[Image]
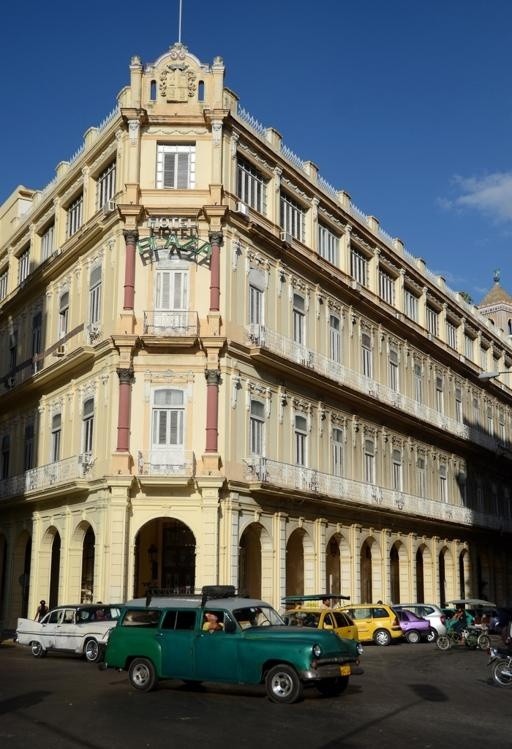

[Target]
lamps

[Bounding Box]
[147,544,159,561]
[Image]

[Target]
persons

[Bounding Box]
[321,598,331,609]
[35,600,50,623]
[93,601,109,622]
[375,601,386,616]
[452,606,468,639]
[202,611,224,632]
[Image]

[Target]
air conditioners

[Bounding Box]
[4,324,99,391]
[102,200,115,214]
[52,248,61,258]
[279,232,293,245]
[78,452,92,465]
[352,282,362,294]
[235,203,250,217]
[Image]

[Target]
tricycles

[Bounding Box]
[436,626,491,651]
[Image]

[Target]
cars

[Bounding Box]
[389,603,447,643]
[15,603,124,664]
[437,606,511,636]
[281,607,359,643]
[103,585,365,705]
[335,604,402,647]
[385,609,432,644]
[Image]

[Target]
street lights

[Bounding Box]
[475,370,512,380]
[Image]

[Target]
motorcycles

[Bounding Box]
[485,645,512,688]
